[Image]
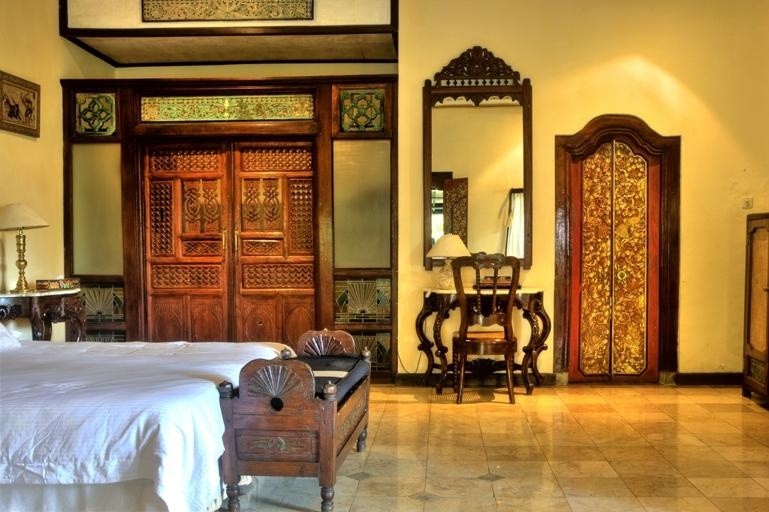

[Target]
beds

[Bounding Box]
[0,325,296,512]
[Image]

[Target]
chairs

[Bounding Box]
[450,252,520,406]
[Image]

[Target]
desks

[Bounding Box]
[416,288,551,395]
[0,289,86,343]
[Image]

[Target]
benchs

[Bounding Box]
[219,329,371,512]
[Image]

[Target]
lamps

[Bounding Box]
[425,233,472,289]
[0,205,49,293]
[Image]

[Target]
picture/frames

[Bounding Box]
[0,68,41,140]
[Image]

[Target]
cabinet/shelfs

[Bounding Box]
[554,115,682,388]
[741,212,768,410]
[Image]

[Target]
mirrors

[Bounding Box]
[422,46,533,272]
